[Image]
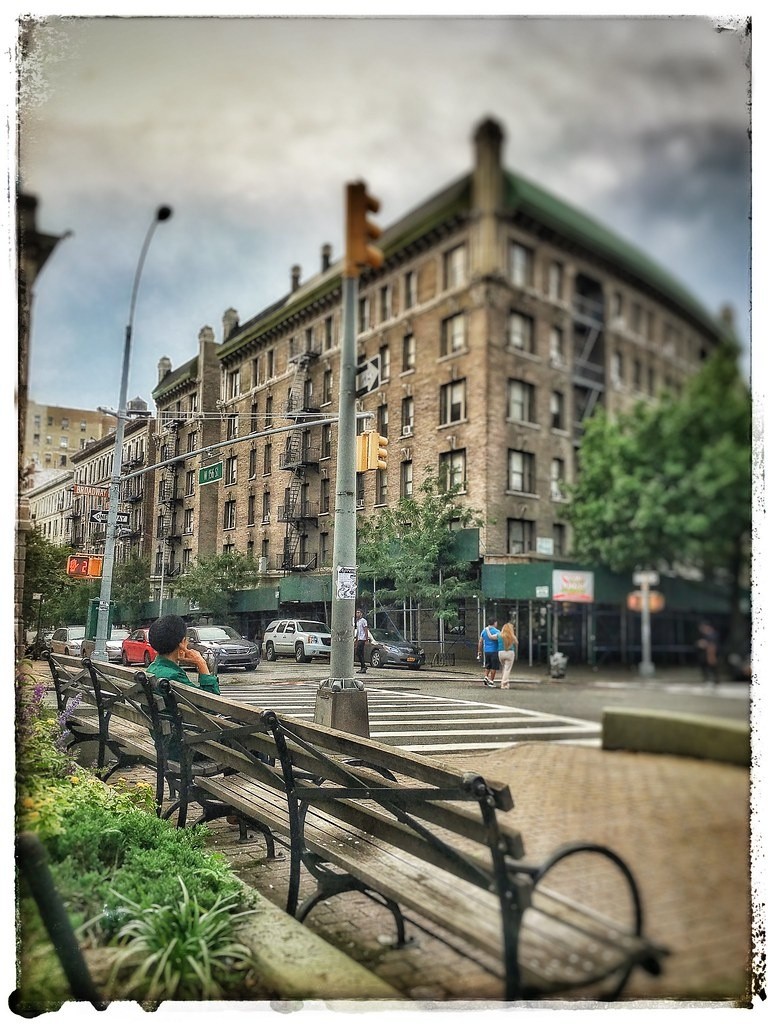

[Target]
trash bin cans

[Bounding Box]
[548,652,569,678]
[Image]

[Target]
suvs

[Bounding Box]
[262,619,332,663]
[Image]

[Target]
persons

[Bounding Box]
[698,621,719,682]
[478,617,500,685]
[141,613,270,824]
[486,623,519,689]
[356,608,368,673]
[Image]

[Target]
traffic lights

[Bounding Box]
[67,555,90,576]
[367,433,388,470]
[346,181,385,268]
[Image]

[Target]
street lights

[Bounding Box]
[91,205,174,664]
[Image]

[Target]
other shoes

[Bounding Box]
[356,668,366,673]
[483,678,488,686]
[501,683,509,690]
[226,815,238,824]
[489,682,496,688]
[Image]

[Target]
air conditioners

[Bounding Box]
[185,527,192,533]
[357,499,364,508]
[403,425,413,436]
[264,515,270,521]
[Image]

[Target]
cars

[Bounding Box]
[121,629,158,667]
[354,628,425,670]
[50,628,86,656]
[180,626,260,672]
[80,630,132,661]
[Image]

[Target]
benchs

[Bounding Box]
[41,650,672,1000]
